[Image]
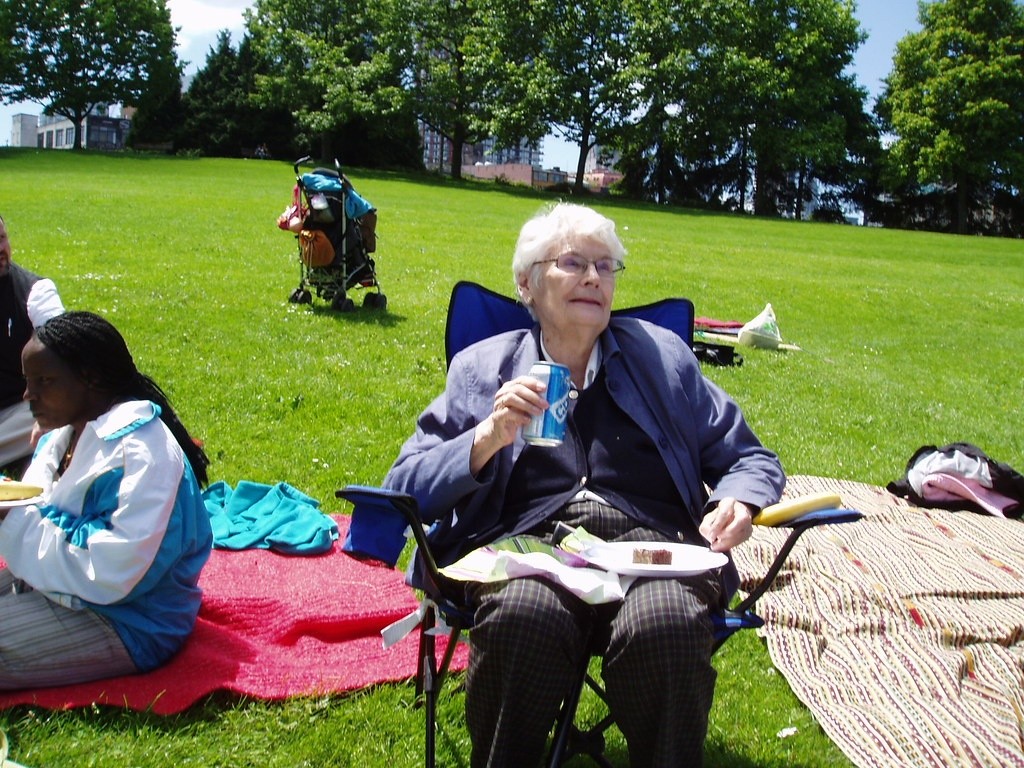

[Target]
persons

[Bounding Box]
[0,216,66,467]
[0,311,213,691]
[380,205,787,768]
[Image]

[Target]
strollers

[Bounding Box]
[288,156,388,314]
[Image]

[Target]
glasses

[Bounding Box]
[533,251,625,279]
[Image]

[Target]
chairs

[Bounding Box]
[336,280,863,768]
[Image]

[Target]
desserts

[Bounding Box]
[632,547,672,565]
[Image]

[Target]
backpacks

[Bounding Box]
[692,340,744,367]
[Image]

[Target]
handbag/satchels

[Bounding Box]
[297,228,335,268]
[275,184,308,232]
[738,303,781,349]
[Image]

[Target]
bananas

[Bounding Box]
[752,491,840,526]
[0,482,43,501]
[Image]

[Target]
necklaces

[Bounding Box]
[64,445,72,470]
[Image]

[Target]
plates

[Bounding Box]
[0,496,43,510]
[581,541,729,576]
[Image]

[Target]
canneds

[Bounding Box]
[520,361,570,447]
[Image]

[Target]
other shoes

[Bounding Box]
[359,278,379,287]
